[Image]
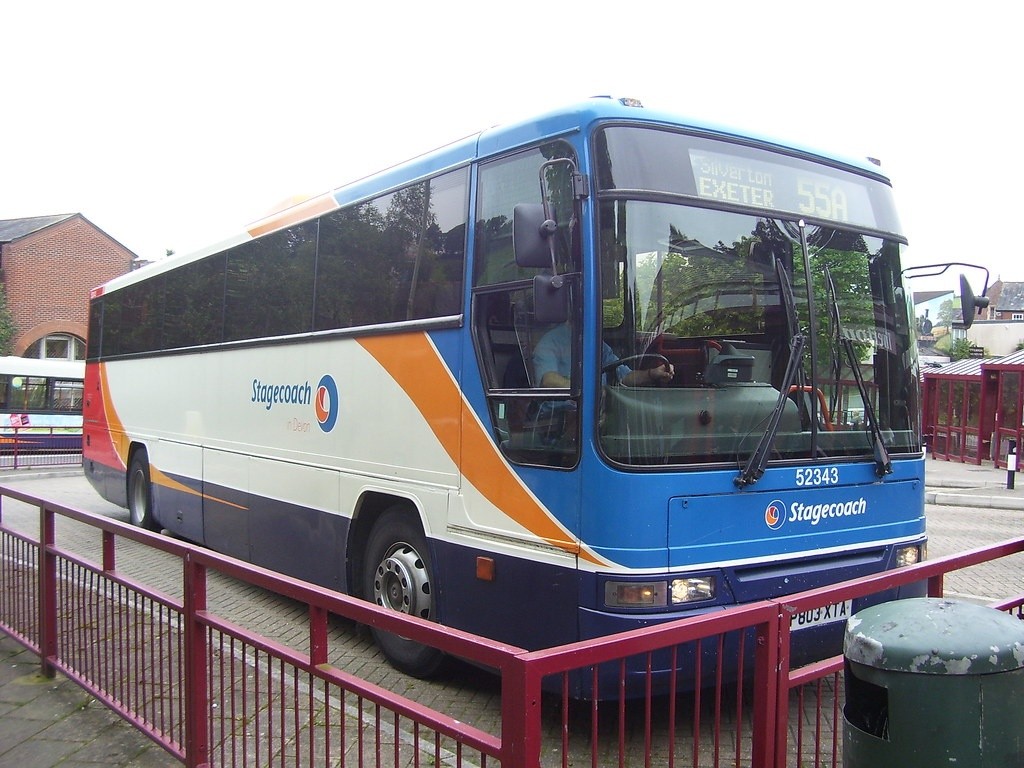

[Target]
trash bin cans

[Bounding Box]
[843,597,1024,768]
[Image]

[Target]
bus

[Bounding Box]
[82,95,990,706]
[0,356,86,452]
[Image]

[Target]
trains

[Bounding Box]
[915,308,934,347]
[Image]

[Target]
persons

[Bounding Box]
[531,296,676,449]
[850,410,863,431]
